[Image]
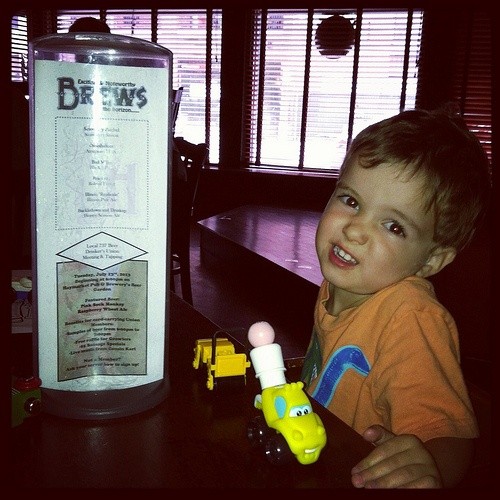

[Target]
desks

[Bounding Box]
[0,290,377,500]
[197,203,324,292]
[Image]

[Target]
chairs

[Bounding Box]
[171,137,210,306]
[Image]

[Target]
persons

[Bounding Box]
[300,109,491,488]
[69,17,113,63]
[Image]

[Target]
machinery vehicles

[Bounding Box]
[191,328,249,395]
